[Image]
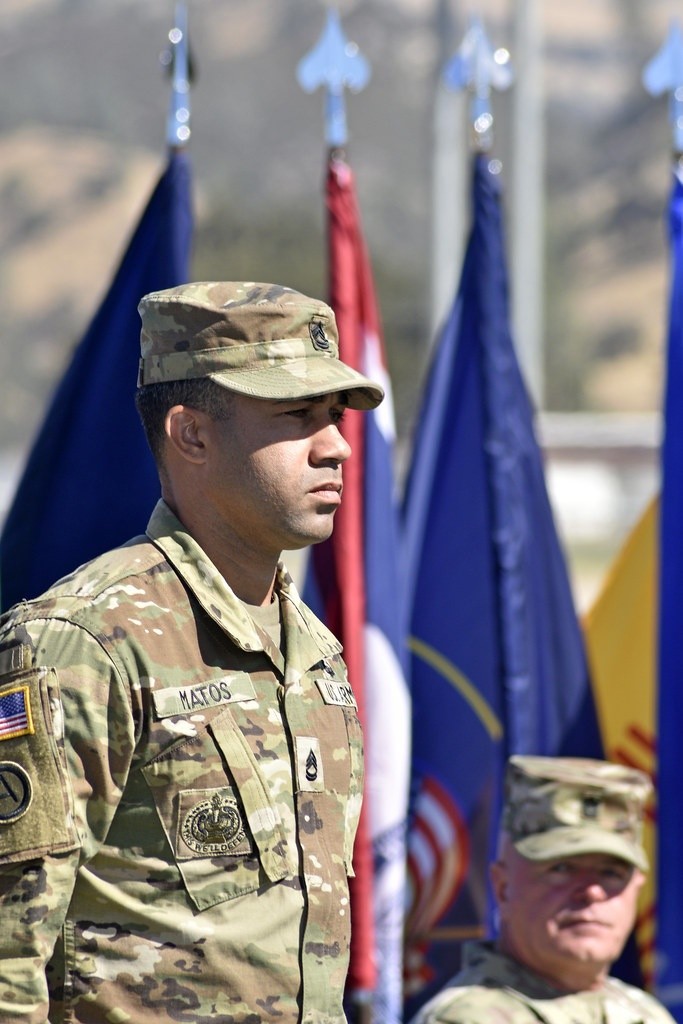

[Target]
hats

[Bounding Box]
[500,754,654,874]
[136,280,385,412]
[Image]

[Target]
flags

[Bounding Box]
[0,160,193,614]
[395,156,645,1024]
[325,150,414,1024]
[582,172,683,1024]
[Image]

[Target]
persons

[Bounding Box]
[0,279,385,1024]
[408,753,677,1024]
